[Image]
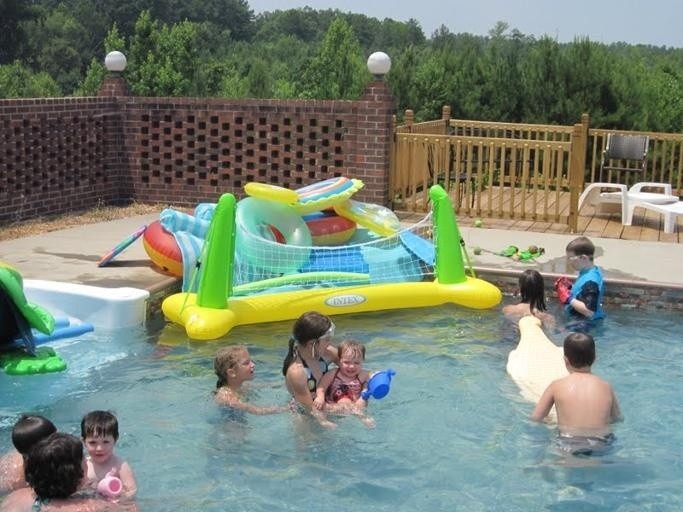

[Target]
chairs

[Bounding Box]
[424,142,488,209]
[575,133,682,234]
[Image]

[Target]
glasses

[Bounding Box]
[306,315,335,343]
[566,255,580,262]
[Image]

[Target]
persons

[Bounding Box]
[279,310,360,439]
[552,236,605,336]
[0,412,58,492]
[527,331,624,459]
[0,430,139,512]
[501,267,558,335]
[311,336,378,432]
[206,343,298,424]
[71,409,140,503]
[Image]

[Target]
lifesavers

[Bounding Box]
[505,317,569,417]
[233,177,401,272]
[142,220,183,278]
